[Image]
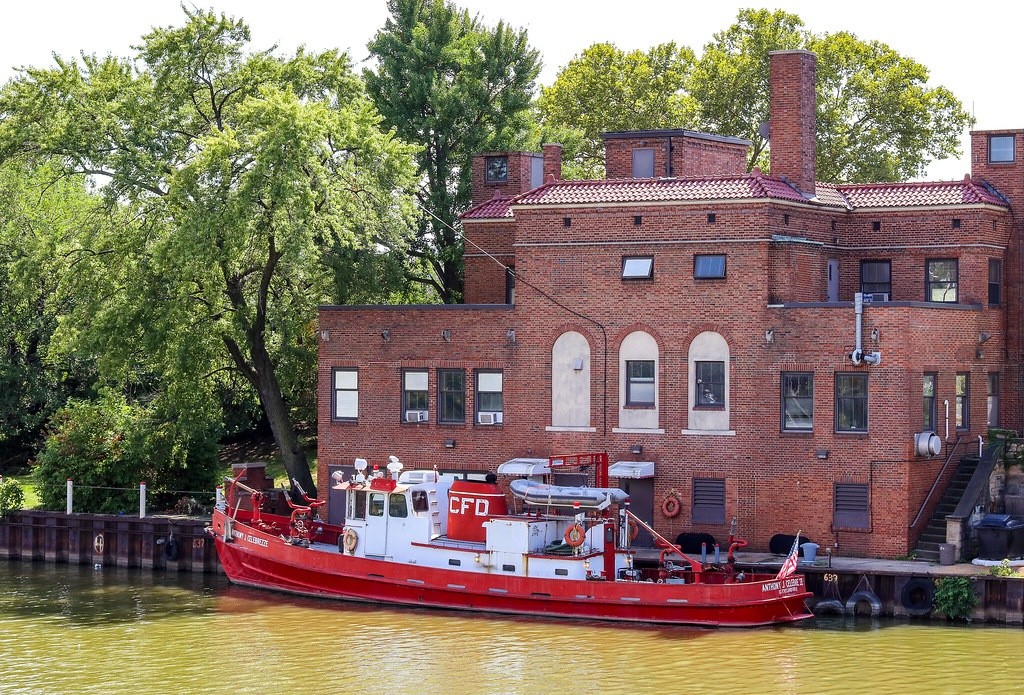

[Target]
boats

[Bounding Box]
[200,451,822,634]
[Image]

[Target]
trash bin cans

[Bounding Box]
[974,514,1024,560]
[938,543,956,564]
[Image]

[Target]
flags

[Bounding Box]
[776,532,798,580]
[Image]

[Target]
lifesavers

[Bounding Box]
[565,525,585,546]
[847,592,882,618]
[900,575,936,618]
[627,519,639,542]
[813,601,844,615]
[342,529,357,550]
[661,498,680,517]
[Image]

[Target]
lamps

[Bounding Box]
[816,450,829,459]
[444,440,456,448]
[630,446,643,454]
